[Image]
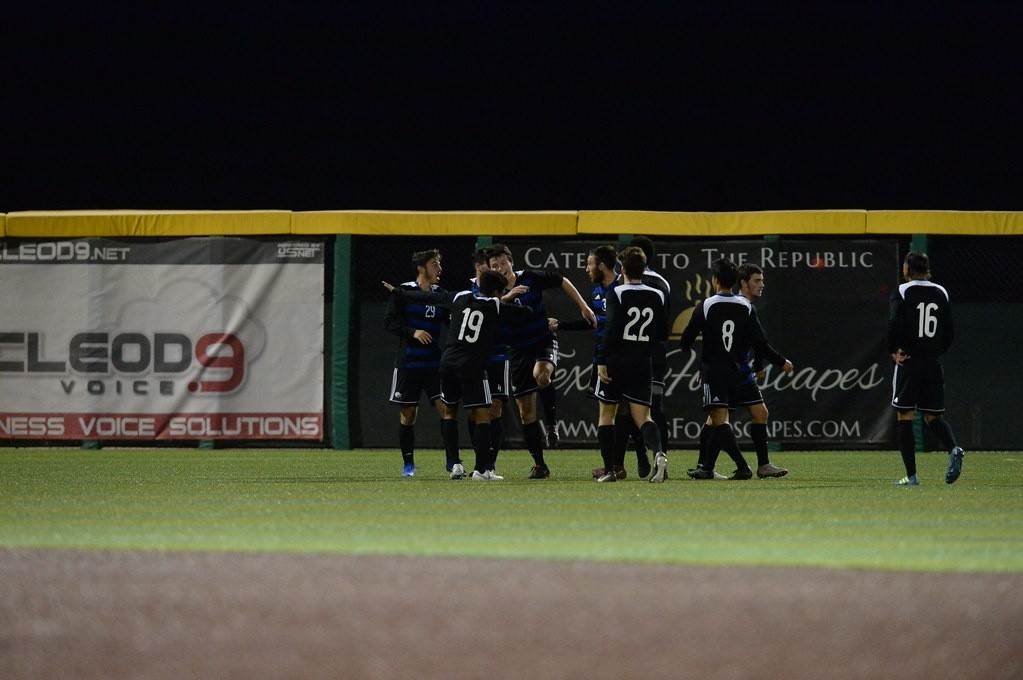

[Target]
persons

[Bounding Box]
[377,236,796,482]
[890,250,965,488]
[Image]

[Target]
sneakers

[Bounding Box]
[649,451,668,483]
[401,463,415,477]
[446,465,466,477]
[594,471,616,482]
[687,468,714,480]
[894,476,919,486]
[637,453,651,478]
[450,464,464,480]
[728,468,753,480]
[472,469,504,482]
[757,463,788,479]
[468,472,474,477]
[592,468,627,480]
[527,464,550,479]
[713,471,727,480]
[946,446,964,484]
